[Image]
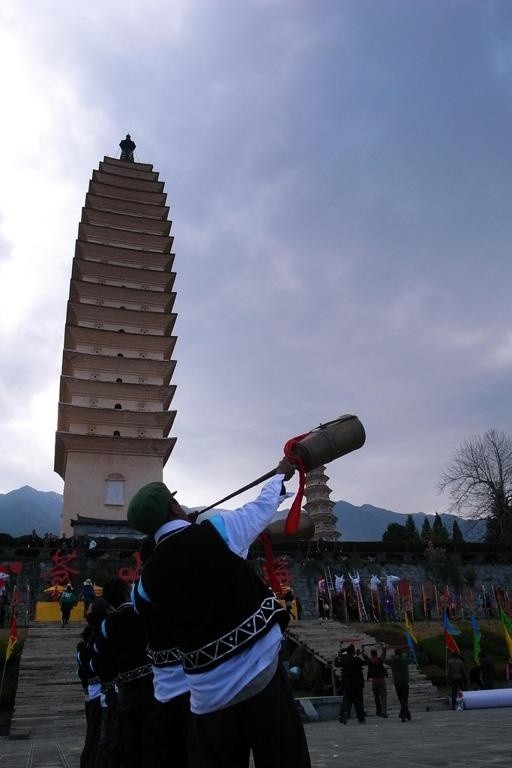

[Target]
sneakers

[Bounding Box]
[340,710,412,726]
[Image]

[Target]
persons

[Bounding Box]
[334,644,368,724]
[447,651,497,710]
[383,644,416,723]
[284,586,296,620]
[75,536,190,768]
[58,583,78,628]
[127,456,315,766]
[361,643,389,719]
[297,596,302,620]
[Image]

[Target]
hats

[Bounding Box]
[126,482,178,534]
